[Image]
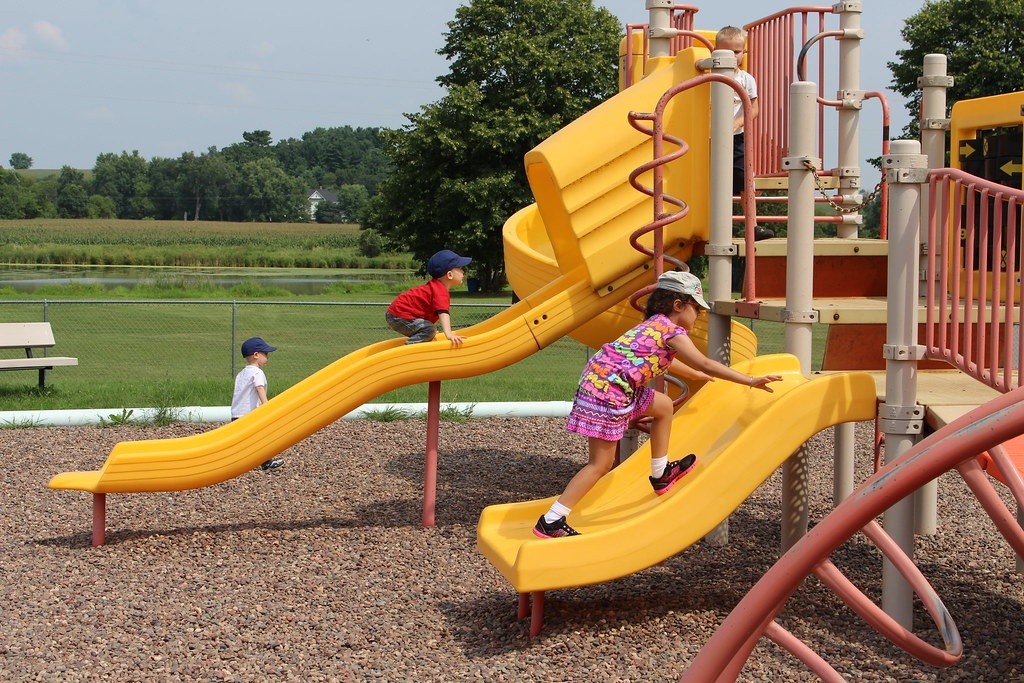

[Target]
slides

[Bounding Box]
[46,29,751,494]
[473,354,878,592]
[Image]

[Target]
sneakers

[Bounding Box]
[532,514,581,539]
[261,457,285,473]
[755,226,774,241]
[649,454,697,495]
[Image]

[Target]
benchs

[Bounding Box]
[0,322,78,390]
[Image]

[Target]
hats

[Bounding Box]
[427,250,472,278]
[656,271,711,309]
[241,337,278,358]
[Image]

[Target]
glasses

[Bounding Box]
[681,301,702,310]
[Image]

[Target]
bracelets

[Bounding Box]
[749,376,753,389]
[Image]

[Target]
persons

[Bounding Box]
[386,249,473,350]
[714,25,769,241]
[230,337,285,470]
[532,271,783,540]
